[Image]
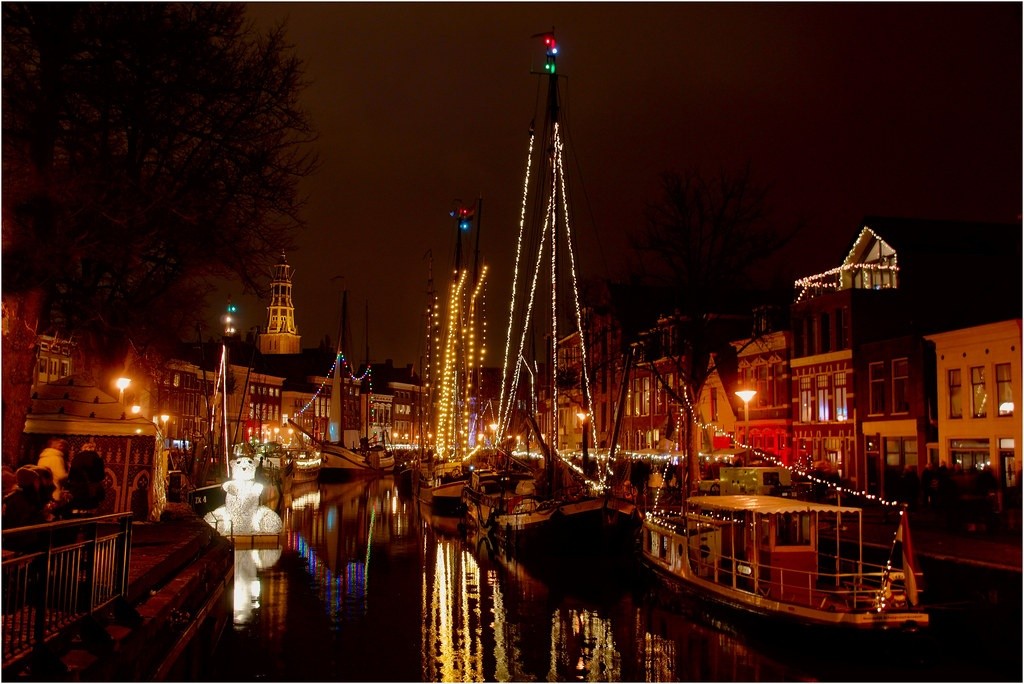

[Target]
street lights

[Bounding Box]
[116,378,131,404]
[734,385,759,465]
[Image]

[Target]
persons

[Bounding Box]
[2,452,108,622]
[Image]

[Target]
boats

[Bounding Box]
[260,444,322,485]
[638,494,929,637]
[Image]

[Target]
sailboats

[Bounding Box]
[319,287,395,484]
[397,30,650,559]
[191,341,230,520]
